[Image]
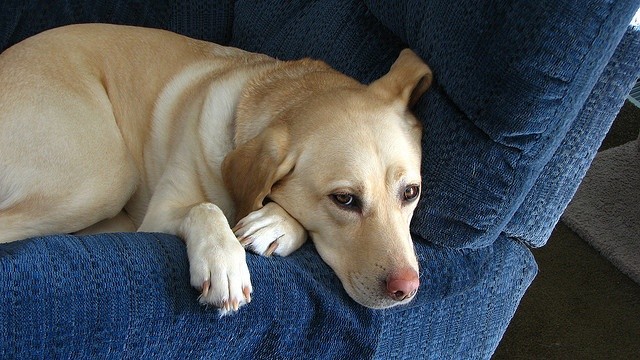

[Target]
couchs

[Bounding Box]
[0,0,639,359]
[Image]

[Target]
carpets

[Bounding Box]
[559,138,636,283]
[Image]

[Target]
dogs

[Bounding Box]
[0,22,434,318]
